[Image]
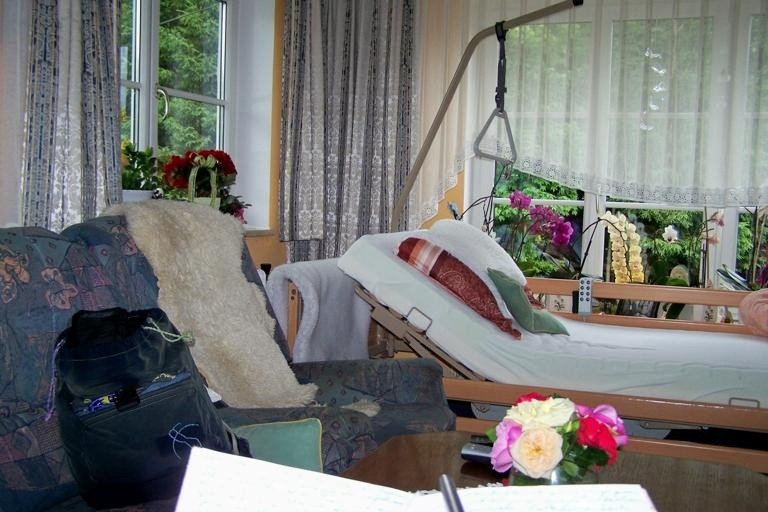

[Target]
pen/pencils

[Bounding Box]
[439,471,464,511]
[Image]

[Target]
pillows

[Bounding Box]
[399,217,543,339]
[229,415,326,475]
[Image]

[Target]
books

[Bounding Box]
[172,445,660,512]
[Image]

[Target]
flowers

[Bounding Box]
[117,142,161,187]
[163,143,238,195]
[223,191,248,223]
[490,390,627,485]
[486,190,576,277]
[655,205,724,288]
[568,204,644,283]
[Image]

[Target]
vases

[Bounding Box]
[540,295,572,315]
[680,303,723,324]
[185,197,220,209]
[121,189,154,202]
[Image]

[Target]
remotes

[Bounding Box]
[459,443,492,463]
[469,433,496,445]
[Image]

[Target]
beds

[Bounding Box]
[336,217,767,475]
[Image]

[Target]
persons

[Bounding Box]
[737,286,768,335]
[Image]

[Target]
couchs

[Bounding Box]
[0,198,457,511]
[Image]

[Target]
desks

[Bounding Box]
[332,430,767,512]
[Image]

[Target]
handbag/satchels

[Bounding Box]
[53,307,253,512]
